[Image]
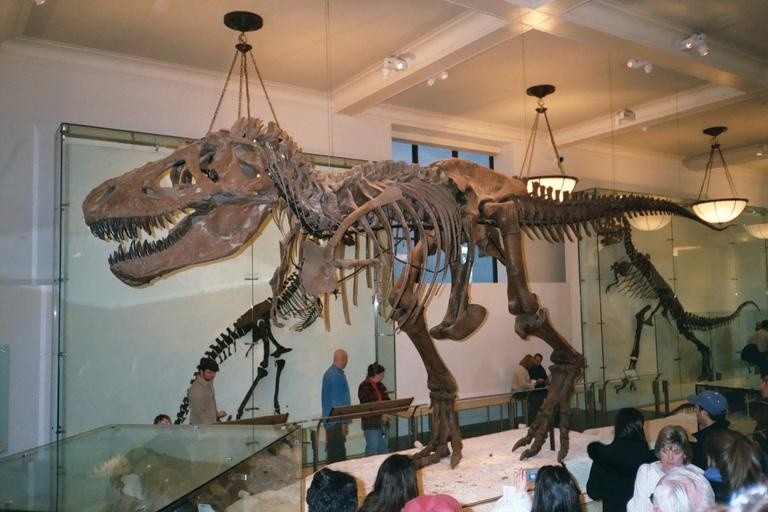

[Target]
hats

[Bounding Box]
[687,390,728,416]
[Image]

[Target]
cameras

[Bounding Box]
[523,469,538,481]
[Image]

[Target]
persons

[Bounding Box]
[511,353,548,426]
[586,319,768,512]
[189,357,227,424]
[358,362,390,457]
[142,414,199,512]
[322,349,352,462]
[358,454,419,512]
[531,464,581,512]
[306,467,358,512]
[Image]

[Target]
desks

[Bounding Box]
[695,375,768,418]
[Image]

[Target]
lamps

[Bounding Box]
[744,223,768,240]
[691,126,748,224]
[518,84,578,190]
[622,194,682,232]
